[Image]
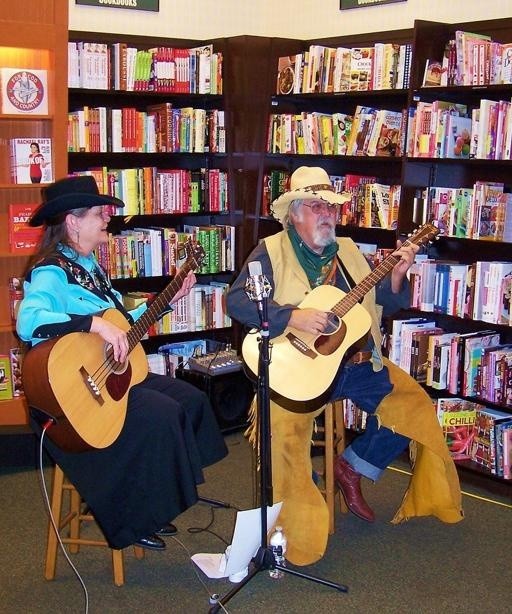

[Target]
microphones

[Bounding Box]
[246,258,276,328]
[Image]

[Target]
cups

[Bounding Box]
[225,545,248,583]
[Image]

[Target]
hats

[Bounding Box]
[269,166,352,225]
[26,176,125,228]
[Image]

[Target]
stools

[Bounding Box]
[44,464,144,586]
[311,400,349,535]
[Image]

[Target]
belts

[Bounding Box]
[349,352,372,364]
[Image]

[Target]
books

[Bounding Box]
[269,99,512,160]
[406,254,512,326]
[10,279,24,320]
[355,243,395,266]
[121,282,232,340]
[261,173,401,230]
[147,339,229,379]
[277,31,512,94]
[68,43,222,95]
[9,205,45,255]
[93,225,235,280]
[0,348,24,400]
[10,139,52,184]
[68,103,226,153]
[343,398,512,481]
[70,167,227,216]
[414,181,512,243]
[391,318,512,408]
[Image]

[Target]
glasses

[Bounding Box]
[302,201,338,215]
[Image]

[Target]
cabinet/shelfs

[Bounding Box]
[252,17,511,496]
[68,34,238,347]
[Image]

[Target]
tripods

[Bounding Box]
[203,290,351,614]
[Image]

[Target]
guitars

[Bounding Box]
[21,237,206,453]
[242,221,441,414]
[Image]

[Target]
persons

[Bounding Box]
[226,167,433,525]
[17,174,231,550]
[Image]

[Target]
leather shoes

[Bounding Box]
[155,523,177,536]
[333,455,376,522]
[134,535,167,550]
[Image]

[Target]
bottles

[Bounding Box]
[267,526,288,579]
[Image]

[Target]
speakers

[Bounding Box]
[176,368,253,432]
